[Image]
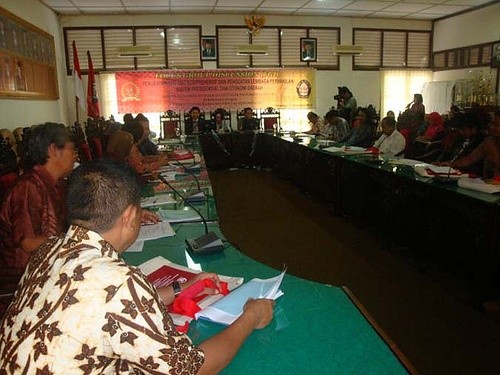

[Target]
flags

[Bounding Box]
[72,41,85,111]
[87,50,100,118]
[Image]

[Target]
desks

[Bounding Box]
[122,131,500,375]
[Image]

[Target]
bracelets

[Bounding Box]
[172,282,181,298]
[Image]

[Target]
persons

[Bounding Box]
[185,107,205,135]
[206,109,231,135]
[239,108,258,131]
[0,160,275,375]
[304,87,500,179]
[0,113,171,293]
[302,40,314,58]
[203,39,215,57]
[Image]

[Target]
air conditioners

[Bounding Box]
[236,45,268,56]
[117,46,152,58]
[332,45,364,56]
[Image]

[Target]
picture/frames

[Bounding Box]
[491,40,500,68]
[299,37,318,62]
[200,36,218,61]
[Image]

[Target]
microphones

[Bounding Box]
[157,136,225,255]
[439,145,469,184]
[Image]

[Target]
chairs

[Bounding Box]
[0,115,115,300]
[352,105,381,137]
[236,107,281,131]
[160,108,231,137]
